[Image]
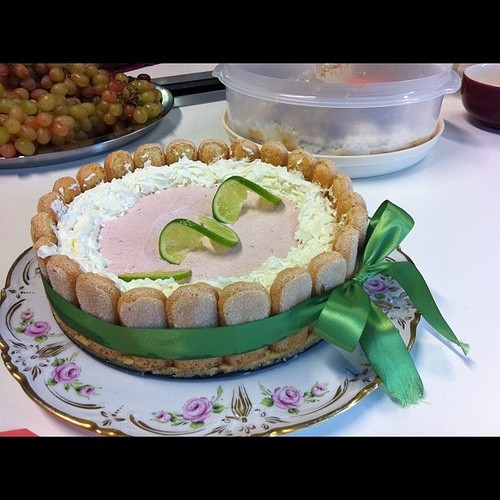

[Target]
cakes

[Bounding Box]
[28,133,371,379]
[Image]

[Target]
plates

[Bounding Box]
[0,216,421,436]
[0,84,174,168]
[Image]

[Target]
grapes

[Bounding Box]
[0,61,164,158]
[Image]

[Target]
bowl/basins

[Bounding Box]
[222,110,445,179]
[460,63,500,128]
[211,64,462,155]
